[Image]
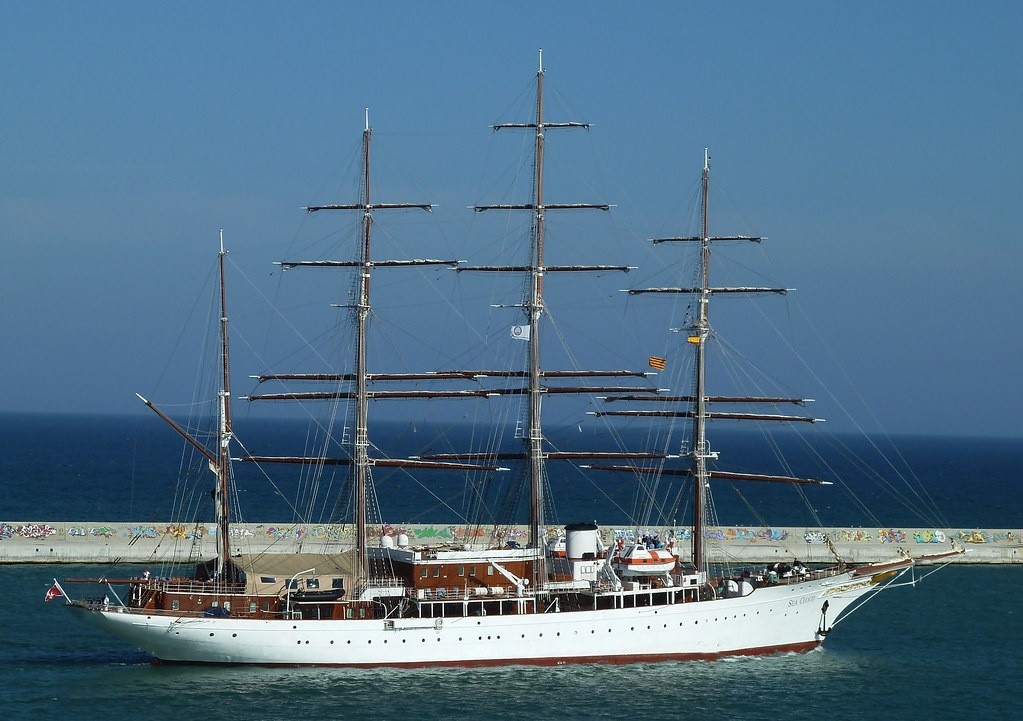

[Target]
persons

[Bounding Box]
[767,558,803,582]
[143,569,150,578]
[643,535,663,548]
[88,594,109,610]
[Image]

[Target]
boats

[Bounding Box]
[614,543,676,576]
[290,587,345,601]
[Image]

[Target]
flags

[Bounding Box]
[649,357,666,371]
[45,586,61,602]
[510,325,530,341]
[688,336,700,344]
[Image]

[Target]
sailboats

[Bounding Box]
[51,51,968,672]
